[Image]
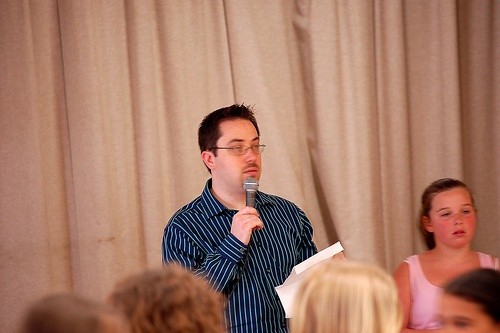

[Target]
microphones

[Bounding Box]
[243,176,259,208]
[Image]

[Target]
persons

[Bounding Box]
[391,178,500,333]
[162,103,345,333]
[440,267,500,333]
[289,260,405,333]
[22,265,229,333]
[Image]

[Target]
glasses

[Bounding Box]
[211,145,266,155]
